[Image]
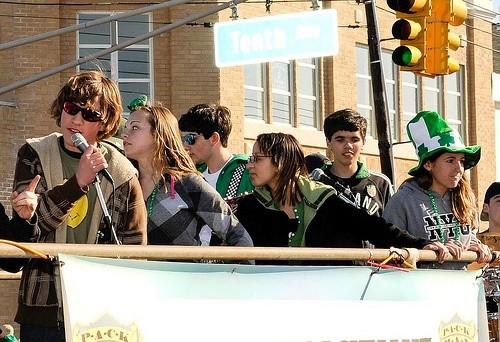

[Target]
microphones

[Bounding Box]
[311,168,352,195]
[71,133,114,184]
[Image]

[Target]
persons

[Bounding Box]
[0,174,41,273]
[14,72,148,342]
[102,94,492,267]
[466,182,500,342]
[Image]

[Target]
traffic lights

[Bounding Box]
[387,0,432,73]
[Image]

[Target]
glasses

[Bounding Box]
[63,101,105,122]
[181,133,204,145]
[250,155,275,163]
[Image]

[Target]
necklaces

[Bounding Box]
[147,180,160,216]
[425,187,459,244]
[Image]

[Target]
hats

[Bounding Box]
[480,182,500,221]
[407,110,482,176]
